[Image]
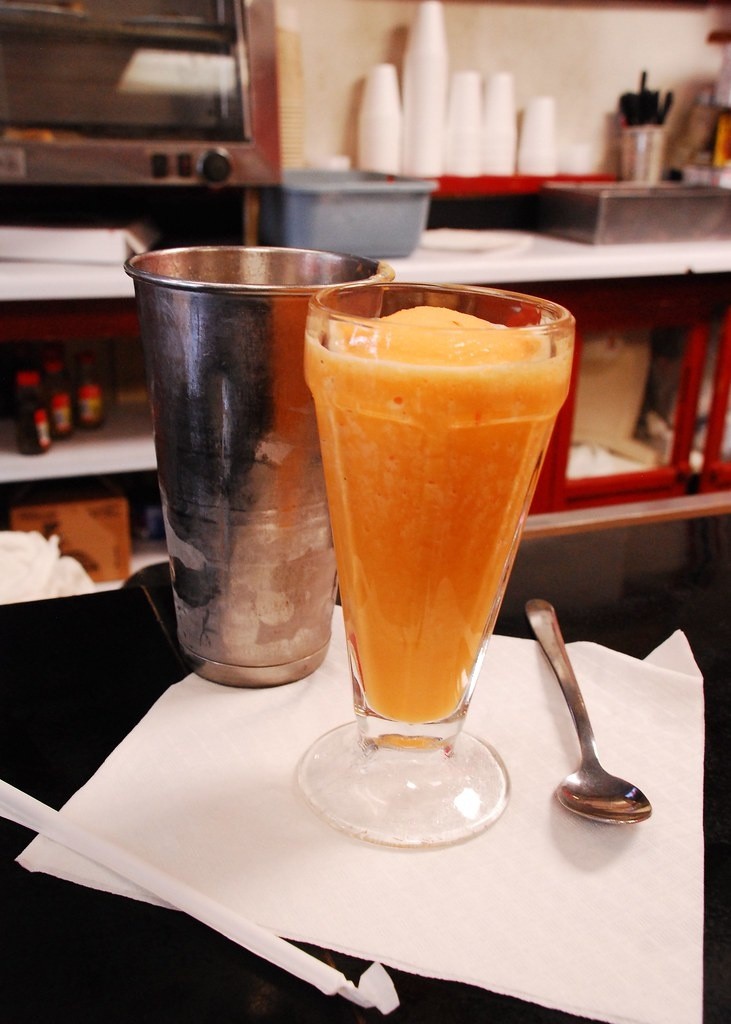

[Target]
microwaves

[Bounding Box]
[0,0,282,190]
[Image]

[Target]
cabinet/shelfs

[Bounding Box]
[382,230,731,541]
[0,215,160,483]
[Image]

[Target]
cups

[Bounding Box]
[297,281,576,846]
[275,27,304,171]
[356,1,557,176]
[123,247,396,689]
[621,125,666,182]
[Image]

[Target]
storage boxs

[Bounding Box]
[283,167,439,258]
[536,180,731,243]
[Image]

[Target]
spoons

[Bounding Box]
[525,598,652,826]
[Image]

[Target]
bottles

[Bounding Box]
[15,349,104,456]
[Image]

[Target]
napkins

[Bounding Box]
[15,628,706,1024]
